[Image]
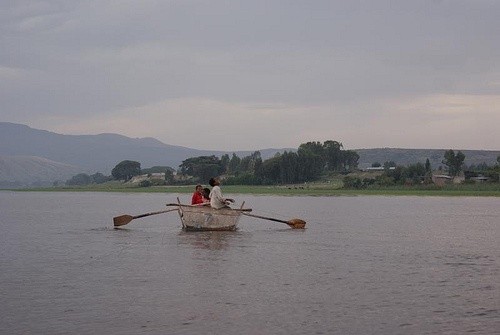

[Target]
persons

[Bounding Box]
[209,176,235,210]
[192,185,203,205]
[201,188,211,203]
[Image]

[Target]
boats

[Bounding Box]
[166,196,253,232]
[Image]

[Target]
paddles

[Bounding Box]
[241,212,306,229]
[113,202,210,226]
